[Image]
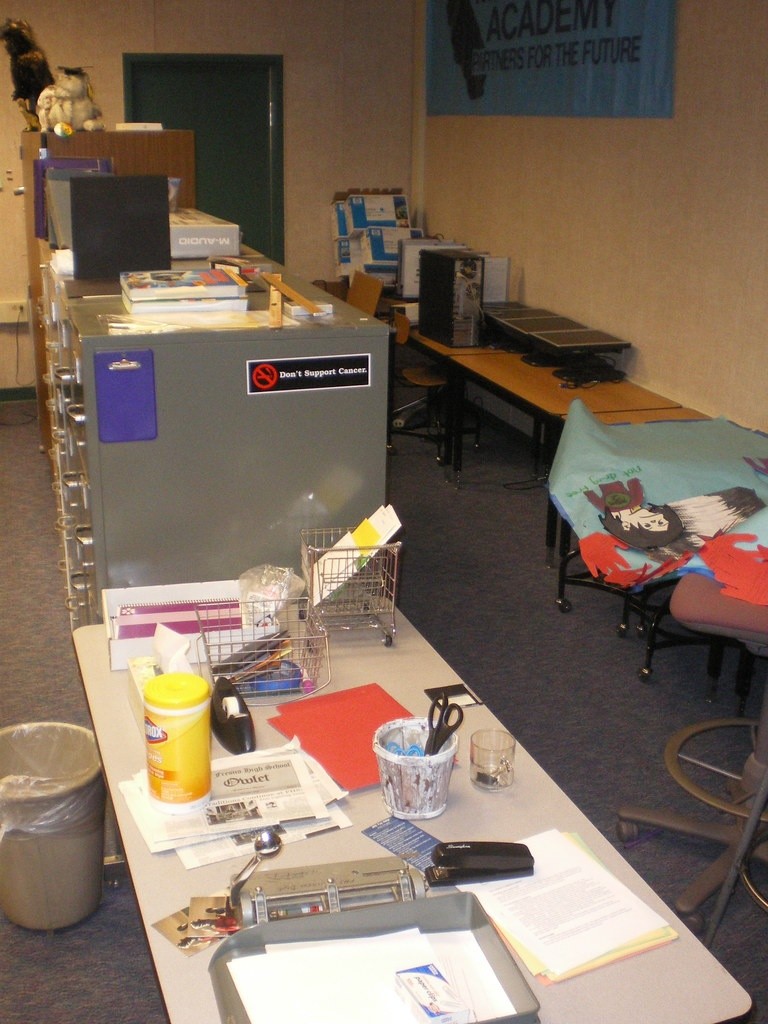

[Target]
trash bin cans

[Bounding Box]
[0,720,108,933]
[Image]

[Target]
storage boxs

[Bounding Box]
[397,964,478,1024]
[334,241,396,279]
[170,209,241,260]
[336,201,349,238]
[361,227,424,271]
[346,193,411,238]
[209,892,542,1024]
[101,582,278,671]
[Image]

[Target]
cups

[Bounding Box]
[469,728,517,794]
[373,717,459,819]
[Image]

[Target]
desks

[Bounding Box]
[72,297,768,1024]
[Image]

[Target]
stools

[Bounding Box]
[614,570,767,935]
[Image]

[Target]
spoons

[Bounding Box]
[231,830,282,886]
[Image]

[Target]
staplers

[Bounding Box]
[425,841,535,886]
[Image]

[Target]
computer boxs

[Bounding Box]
[418,248,485,348]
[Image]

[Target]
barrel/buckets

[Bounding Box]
[143,673,212,810]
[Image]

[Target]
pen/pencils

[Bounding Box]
[214,630,290,671]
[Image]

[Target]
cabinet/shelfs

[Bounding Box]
[22,128,198,449]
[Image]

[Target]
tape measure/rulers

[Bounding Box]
[267,271,282,328]
[262,272,324,314]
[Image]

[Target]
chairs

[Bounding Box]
[393,309,466,470]
[348,269,383,316]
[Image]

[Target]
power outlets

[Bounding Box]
[0,300,29,323]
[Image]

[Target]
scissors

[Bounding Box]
[426,692,463,754]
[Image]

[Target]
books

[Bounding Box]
[116,597,241,640]
[212,629,293,683]
[119,270,250,315]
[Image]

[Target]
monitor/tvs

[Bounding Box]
[482,301,631,382]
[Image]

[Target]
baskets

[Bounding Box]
[298,528,401,638]
[194,596,332,706]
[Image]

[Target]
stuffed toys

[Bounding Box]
[37,66,104,133]
